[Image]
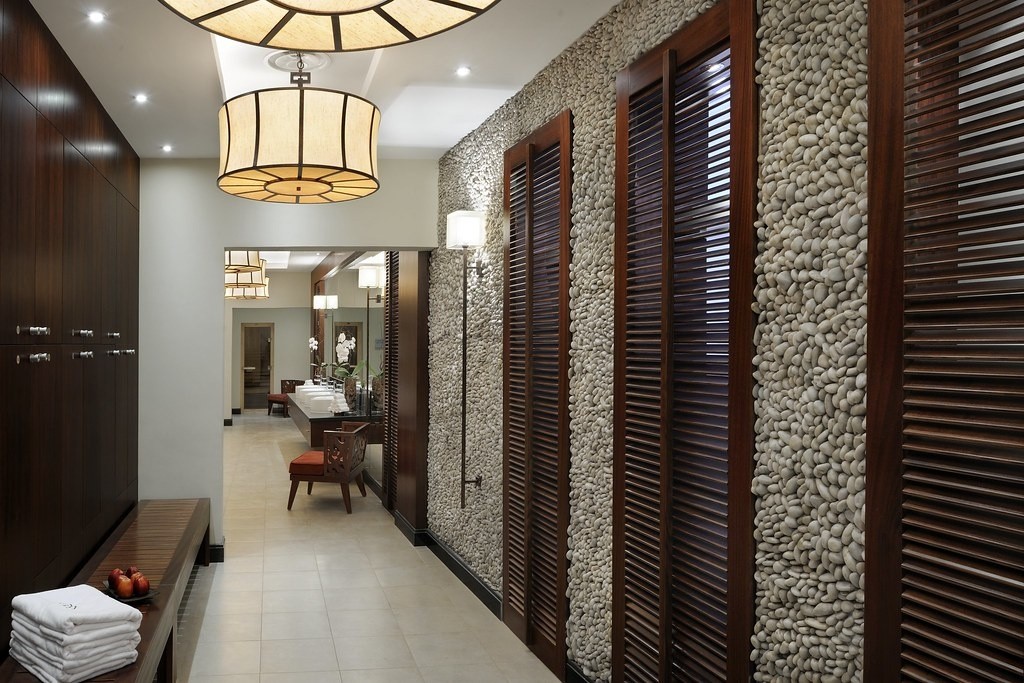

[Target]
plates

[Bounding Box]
[102,580,160,602]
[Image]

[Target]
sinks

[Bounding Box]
[310,395,334,413]
[295,385,320,399]
[299,388,329,403]
[244,367,255,372]
[305,392,333,408]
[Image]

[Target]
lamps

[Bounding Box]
[378,266,386,303]
[216,50,380,206]
[157,0,503,54]
[225,276,270,300]
[326,294,339,318]
[357,266,380,304]
[312,295,326,318]
[225,259,268,288]
[224,250,261,274]
[445,210,487,510]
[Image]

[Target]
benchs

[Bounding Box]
[9,497,213,683]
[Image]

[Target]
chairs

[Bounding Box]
[286,421,371,514]
[267,379,305,418]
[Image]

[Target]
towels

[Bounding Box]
[327,393,350,413]
[8,583,144,683]
[303,379,314,386]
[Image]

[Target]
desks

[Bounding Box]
[285,391,361,448]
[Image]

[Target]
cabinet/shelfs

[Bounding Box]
[0,0,140,683]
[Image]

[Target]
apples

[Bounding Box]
[108,566,149,598]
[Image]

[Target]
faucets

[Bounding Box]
[321,378,327,384]
[335,384,343,392]
[328,381,334,388]
[314,374,321,382]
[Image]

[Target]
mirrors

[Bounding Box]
[314,250,386,412]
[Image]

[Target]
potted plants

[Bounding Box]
[369,355,385,408]
[334,362,365,412]
[309,355,328,384]
[330,360,350,388]
